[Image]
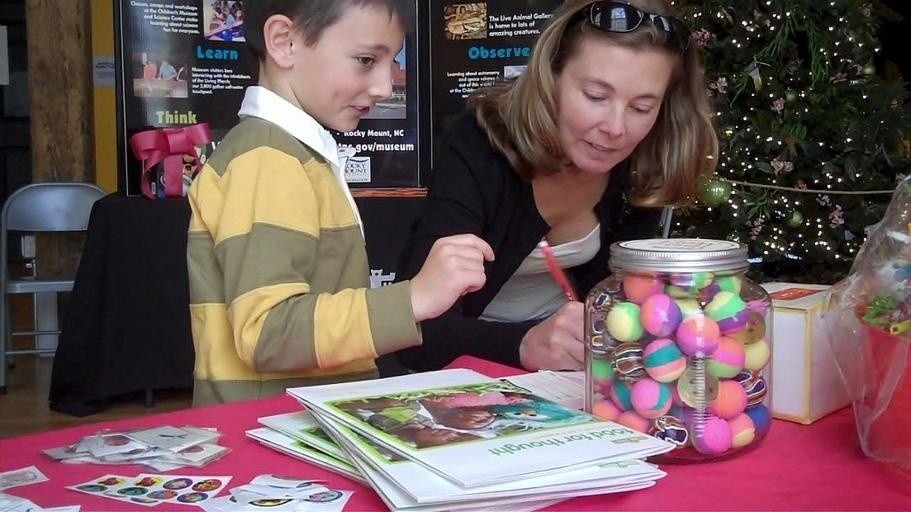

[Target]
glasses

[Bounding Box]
[579,0,693,51]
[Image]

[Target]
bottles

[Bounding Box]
[580,230,769,453]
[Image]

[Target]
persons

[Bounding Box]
[141,59,157,79]
[156,59,179,81]
[388,0,734,377]
[443,3,486,40]
[223,2,243,36]
[179,0,501,416]
[210,6,226,27]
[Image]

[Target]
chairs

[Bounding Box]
[0,182,108,396]
[48,192,195,407]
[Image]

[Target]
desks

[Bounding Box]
[0,358,911,512]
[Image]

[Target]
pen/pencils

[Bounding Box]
[540,240,578,300]
